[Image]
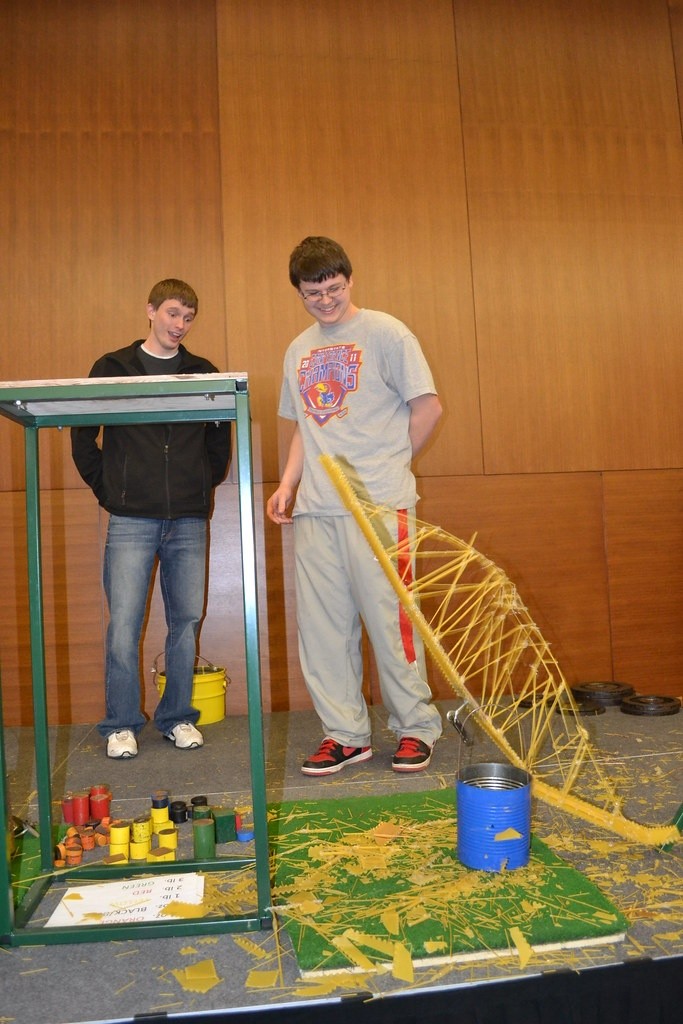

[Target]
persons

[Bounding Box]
[265,234,445,779]
[68,276,234,761]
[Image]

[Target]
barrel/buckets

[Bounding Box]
[152,651,231,726]
[454,704,533,873]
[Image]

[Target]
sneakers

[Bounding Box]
[162,723,204,749]
[301,739,373,776]
[392,737,434,772]
[106,730,138,759]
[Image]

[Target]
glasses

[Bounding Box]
[298,278,347,302]
